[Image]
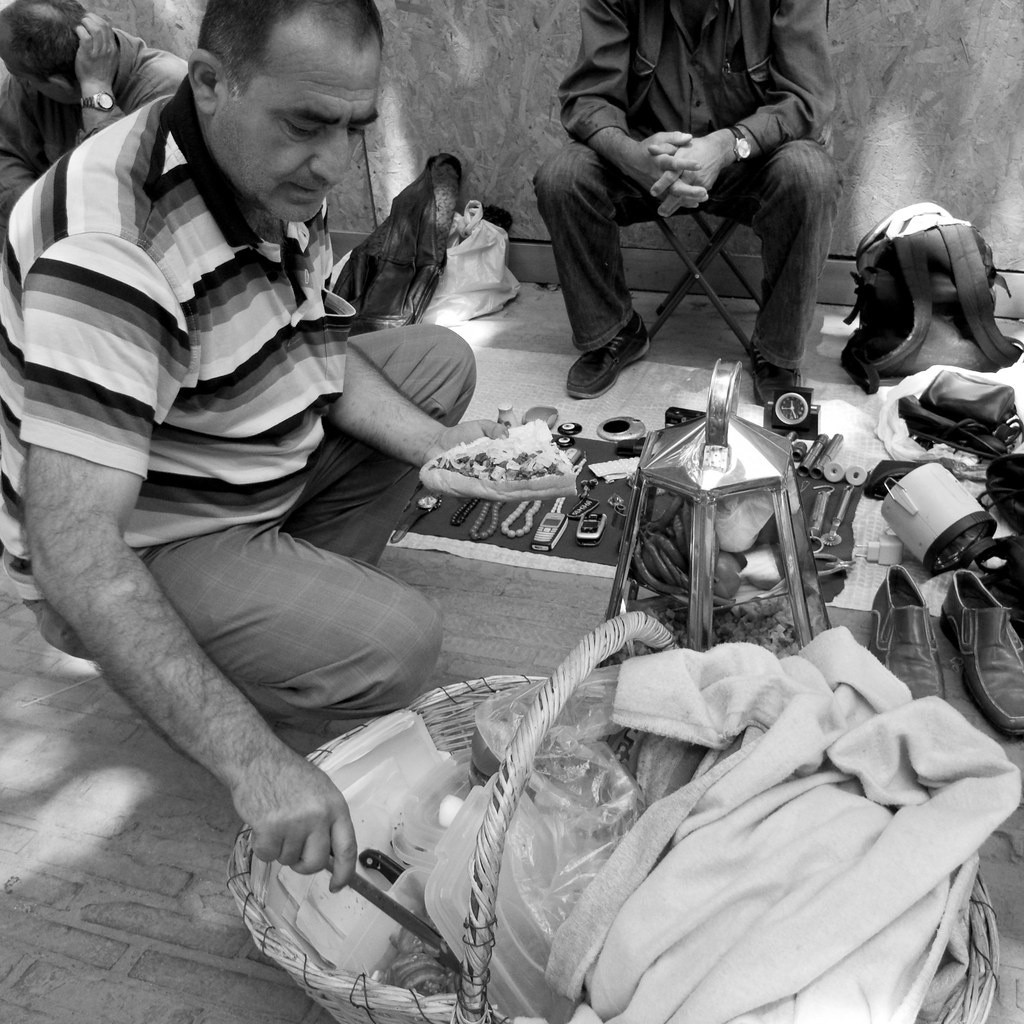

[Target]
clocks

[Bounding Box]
[764,384,822,439]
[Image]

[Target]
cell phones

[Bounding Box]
[531,513,569,552]
[575,512,608,546]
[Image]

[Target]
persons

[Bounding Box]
[0,0,192,247]
[0,0,519,891]
[534,0,837,400]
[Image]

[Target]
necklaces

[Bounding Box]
[550,458,590,513]
[501,500,545,537]
[468,502,505,540]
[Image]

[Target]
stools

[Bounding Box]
[617,202,765,358]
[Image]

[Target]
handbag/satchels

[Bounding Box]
[328,199,521,325]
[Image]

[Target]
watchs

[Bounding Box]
[390,494,443,544]
[81,93,119,113]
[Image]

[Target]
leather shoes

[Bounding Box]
[939,570,1024,738]
[867,563,947,704]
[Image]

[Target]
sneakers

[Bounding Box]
[566,313,649,399]
[750,337,800,405]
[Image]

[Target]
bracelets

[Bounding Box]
[451,499,480,525]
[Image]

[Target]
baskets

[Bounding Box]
[226,609,1000,1024]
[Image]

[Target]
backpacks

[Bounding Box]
[840,203,1024,396]
